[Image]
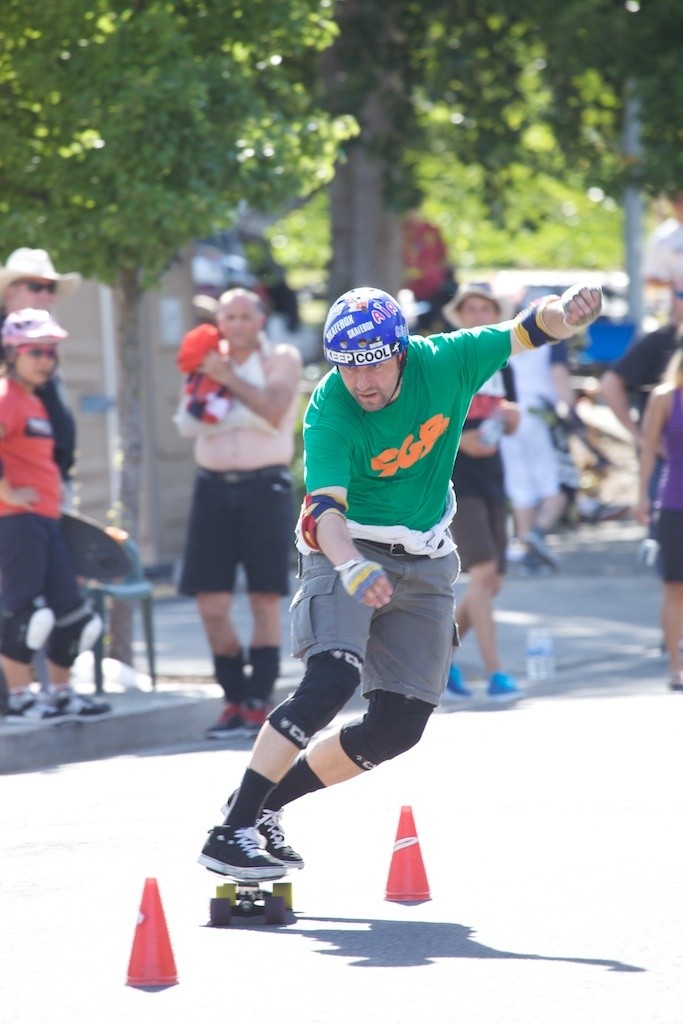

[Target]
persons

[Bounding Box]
[197,281,604,877]
[0,248,683,739]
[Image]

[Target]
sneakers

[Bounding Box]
[241,697,277,737]
[49,689,112,723]
[198,825,286,881]
[0,694,67,725]
[205,702,247,739]
[221,788,303,868]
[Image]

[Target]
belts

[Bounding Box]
[358,539,412,557]
[195,466,288,485]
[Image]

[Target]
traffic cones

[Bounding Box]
[386,805,432,902]
[125,876,179,988]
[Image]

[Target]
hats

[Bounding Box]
[441,280,518,332]
[0,247,75,304]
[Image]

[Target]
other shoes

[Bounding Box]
[445,663,470,701]
[485,672,525,703]
[670,672,683,689]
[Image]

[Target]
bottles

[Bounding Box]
[525,628,556,681]
[480,405,514,445]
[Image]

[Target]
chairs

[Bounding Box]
[30,511,157,697]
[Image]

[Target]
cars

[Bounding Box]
[448,267,630,322]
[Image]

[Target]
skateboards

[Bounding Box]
[58,511,134,581]
[209,875,294,926]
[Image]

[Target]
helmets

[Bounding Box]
[322,288,410,367]
[2,309,68,347]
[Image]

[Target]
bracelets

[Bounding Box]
[332,557,364,580]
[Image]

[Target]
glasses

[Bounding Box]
[24,280,58,294]
[17,344,56,361]
